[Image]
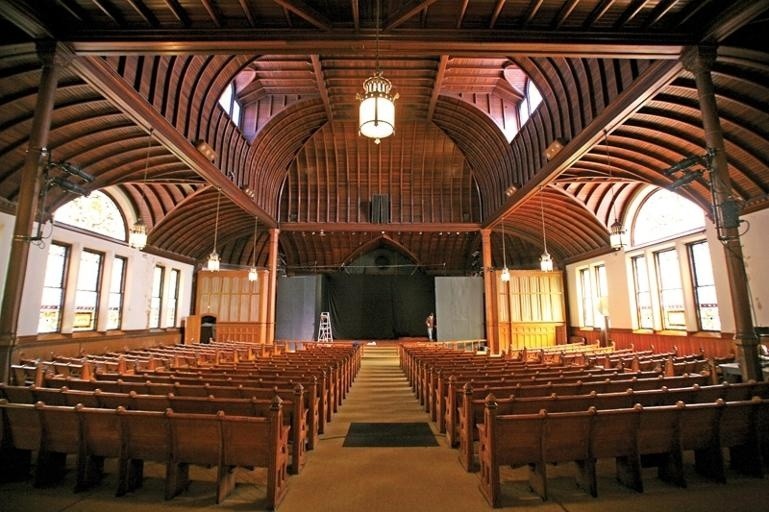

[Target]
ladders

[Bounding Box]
[318,312,333,342]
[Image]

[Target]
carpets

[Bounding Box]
[341,422,440,448]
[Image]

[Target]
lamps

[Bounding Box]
[661,154,739,240]
[29,159,96,244]
[601,130,629,251]
[130,129,153,250]
[355,1,402,146]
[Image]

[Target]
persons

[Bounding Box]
[425,312,437,342]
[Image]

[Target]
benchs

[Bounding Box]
[0,339,363,512]
[399,339,768,508]
